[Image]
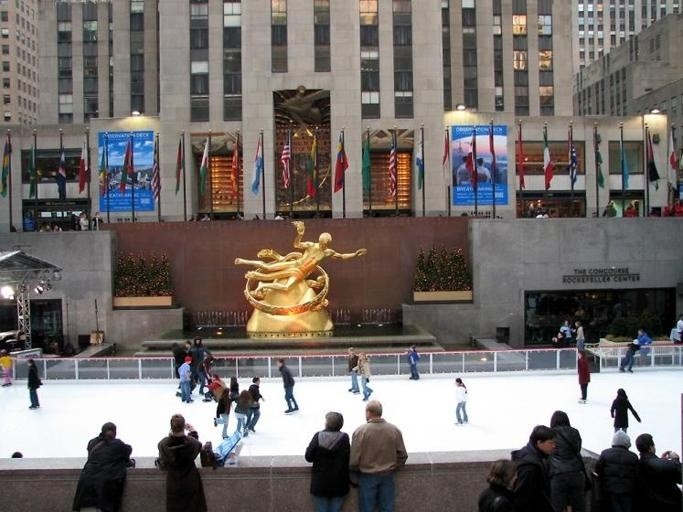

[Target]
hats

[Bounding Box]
[611,431,631,449]
[185,357,193,362]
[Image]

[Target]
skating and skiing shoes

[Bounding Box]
[414,376,420,380]
[352,390,360,393]
[363,399,366,401]
[223,436,230,440]
[250,426,256,433]
[285,408,294,415]
[348,389,354,393]
[214,418,218,427]
[368,390,374,397]
[410,376,415,380]
[454,420,464,427]
[293,408,300,412]
[462,420,469,425]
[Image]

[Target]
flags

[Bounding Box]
[28,144,36,200]
[333,133,349,194]
[78,134,88,195]
[230,137,240,201]
[0,134,11,197]
[119,138,133,193]
[645,126,660,183]
[441,130,451,186]
[542,126,557,190]
[150,135,161,199]
[175,134,185,196]
[199,133,210,197]
[415,127,424,190]
[667,125,678,191]
[619,129,629,193]
[99,140,110,197]
[389,132,397,198]
[306,135,318,199]
[568,124,576,187]
[517,130,525,189]
[593,127,605,189]
[251,132,263,196]
[489,128,496,184]
[280,131,292,189]
[362,133,372,198]
[469,132,476,192]
[56,147,66,200]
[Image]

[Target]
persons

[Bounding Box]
[230,375,240,401]
[276,359,299,413]
[172,343,187,396]
[575,321,586,351]
[72,422,135,512]
[158,413,207,512]
[245,375,264,433]
[548,410,588,512]
[274,213,285,220]
[196,356,214,396]
[560,320,576,344]
[511,424,555,512]
[0,349,14,386]
[203,374,226,402]
[637,329,652,367]
[619,340,641,372]
[634,433,683,512]
[352,352,373,401]
[189,335,209,383]
[184,340,191,354]
[349,400,408,512]
[178,355,194,403]
[552,332,570,349]
[235,221,367,292]
[23,211,102,233]
[454,378,468,424]
[407,345,420,380]
[610,388,641,432]
[677,314,683,344]
[577,350,591,403]
[594,430,639,512]
[478,459,518,512]
[213,388,234,439]
[347,347,360,394]
[234,390,251,437]
[26,359,43,410]
[304,411,351,512]
